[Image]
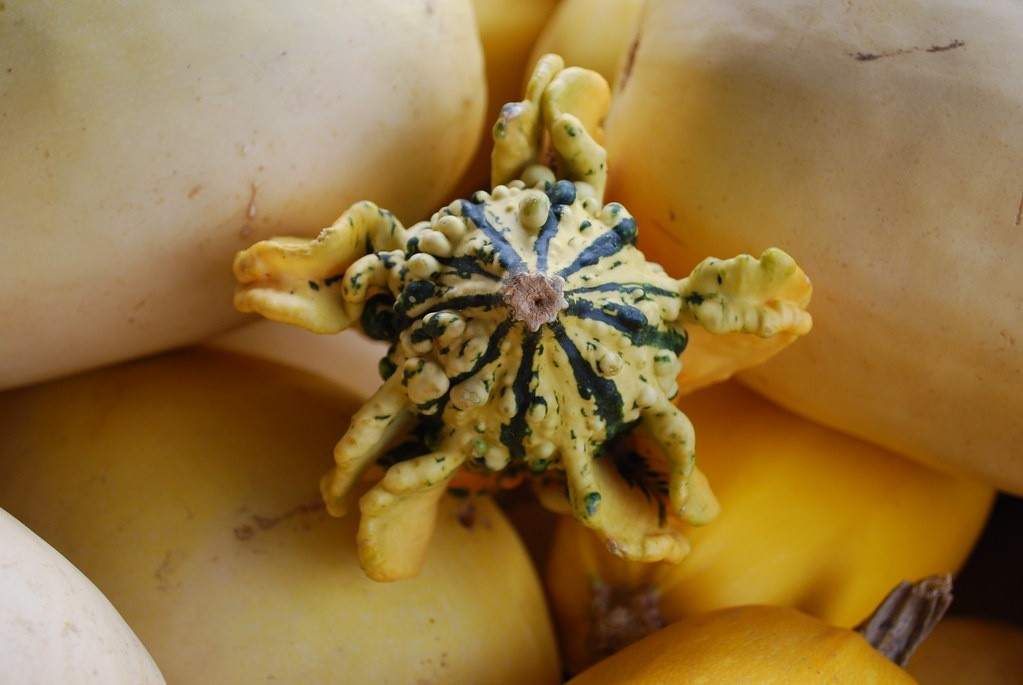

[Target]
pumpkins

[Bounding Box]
[0,0,1023,685]
